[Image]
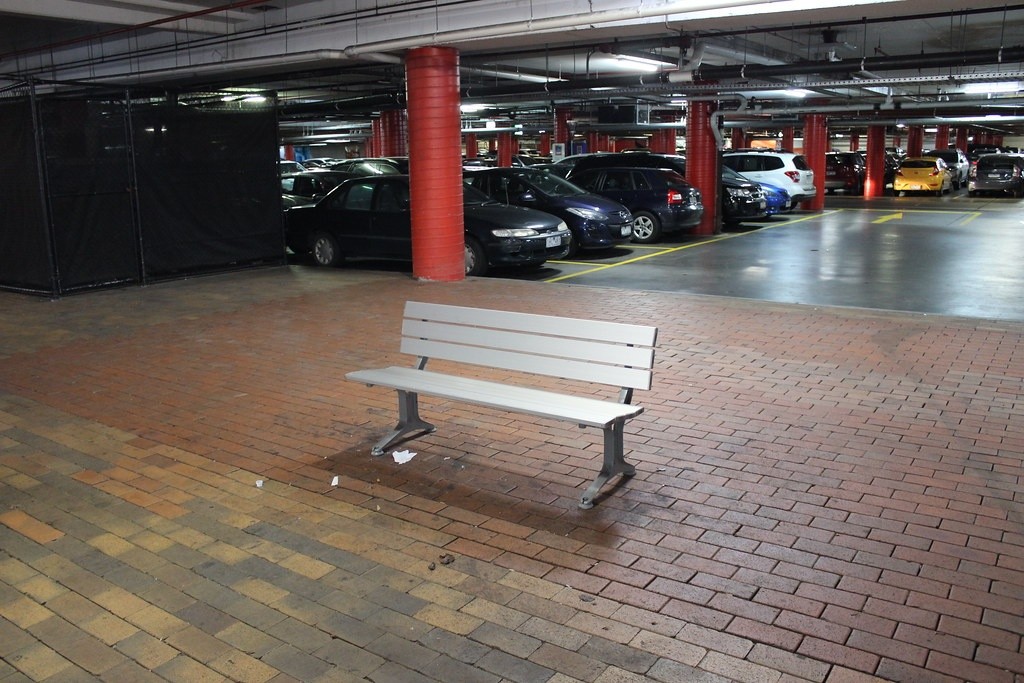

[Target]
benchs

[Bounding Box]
[344,301,658,512]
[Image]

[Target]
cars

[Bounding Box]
[967,154,1024,198]
[282,174,574,277]
[275,146,793,231]
[547,166,704,244]
[893,156,954,198]
[462,165,635,249]
[823,145,1024,196]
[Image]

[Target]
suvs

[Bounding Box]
[720,150,817,212]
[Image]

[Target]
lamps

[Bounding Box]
[963,81,1019,95]
[617,59,657,72]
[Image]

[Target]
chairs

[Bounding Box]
[299,177,313,195]
